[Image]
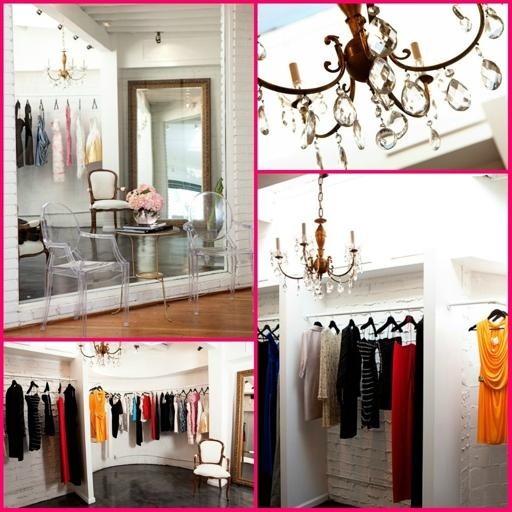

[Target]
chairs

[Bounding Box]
[189,435,232,503]
[8,207,52,298]
[84,162,136,247]
[32,166,253,329]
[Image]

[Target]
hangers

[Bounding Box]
[13,94,106,124]
[5,373,80,398]
[257,309,511,347]
[89,384,211,407]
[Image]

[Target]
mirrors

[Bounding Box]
[230,368,256,490]
[125,75,213,233]
[7,1,237,305]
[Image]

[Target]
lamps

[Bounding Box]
[254,2,510,170]
[40,30,90,90]
[268,172,367,302]
[78,341,125,368]
